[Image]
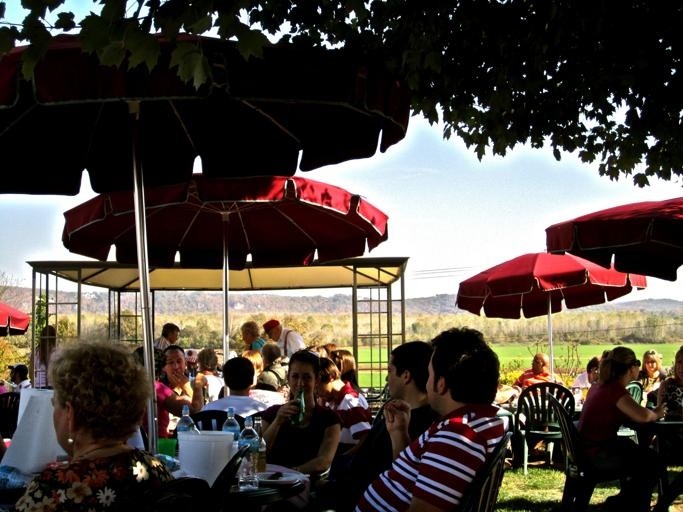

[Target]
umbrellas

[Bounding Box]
[0,303,30,336]
[62,176,389,396]
[455,253,647,381]
[0,33,409,455]
[545,197,683,281]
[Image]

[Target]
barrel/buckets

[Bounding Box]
[177,430,235,488]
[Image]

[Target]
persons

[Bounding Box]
[317,356,372,464]
[199,357,268,418]
[258,344,288,389]
[190,348,225,403]
[638,350,667,392]
[241,321,267,352]
[15,344,175,511]
[352,329,505,512]
[312,342,440,512]
[305,344,337,357]
[219,351,277,401]
[8,364,31,393]
[33,325,61,389]
[331,350,363,393]
[158,345,194,399]
[495,385,522,405]
[512,354,563,393]
[133,347,208,437]
[185,350,197,362]
[152,323,179,349]
[0,380,8,393]
[645,346,683,421]
[263,319,307,365]
[261,350,342,474]
[573,357,600,388]
[577,347,668,512]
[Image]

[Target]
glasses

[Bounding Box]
[455,341,489,365]
[629,360,642,367]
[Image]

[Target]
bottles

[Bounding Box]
[176,405,194,432]
[254,417,266,473]
[289,389,305,425]
[238,416,259,491]
[223,407,240,440]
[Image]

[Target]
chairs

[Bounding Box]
[549,394,632,512]
[454,431,515,512]
[0,391,22,441]
[624,382,644,407]
[515,382,576,478]
[172,408,257,453]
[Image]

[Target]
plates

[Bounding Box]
[257,471,299,485]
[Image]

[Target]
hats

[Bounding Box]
[263,319,280,332]
[8,364,28,376]
